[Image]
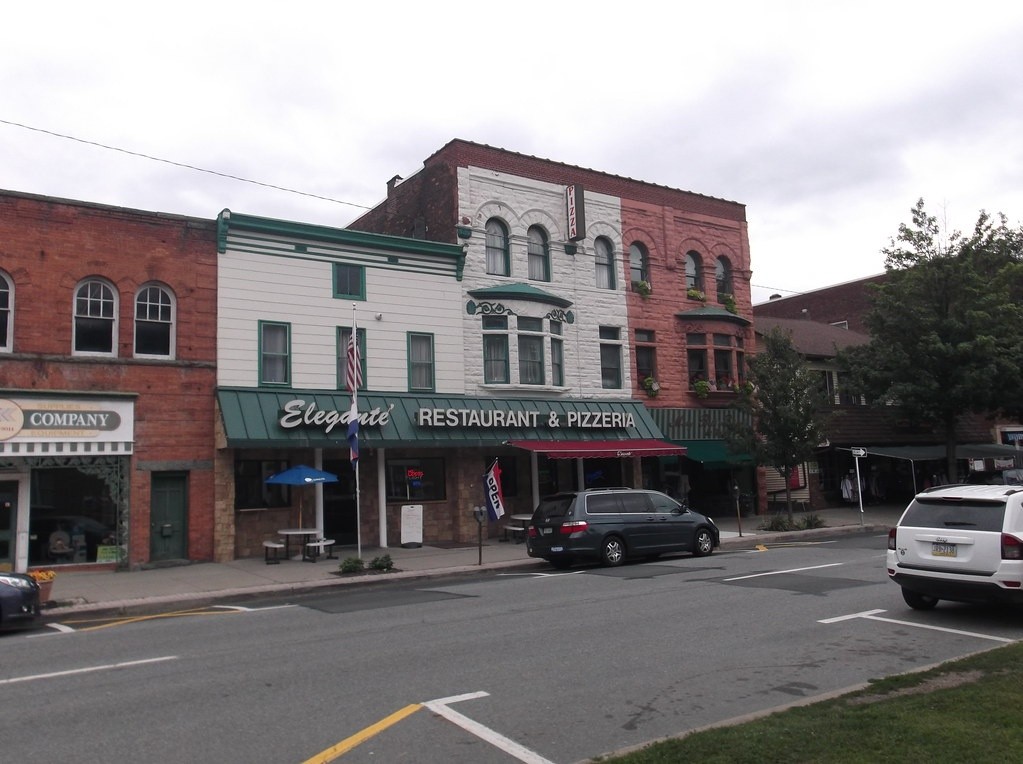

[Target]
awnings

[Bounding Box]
[672,438,759,464]
[506,437,687,460]
[836,441,1021,510]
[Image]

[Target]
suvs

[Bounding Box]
[526,486,721,568]
[886,479,1023,619]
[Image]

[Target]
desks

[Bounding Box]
[277,528,322,560]
[511,512,532,526]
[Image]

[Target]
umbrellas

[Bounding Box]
[264,461,339,528]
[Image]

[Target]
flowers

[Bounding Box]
[27,571,57,581]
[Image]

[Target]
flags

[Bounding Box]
[345,322,362,394]
[485,460,507,524]
[348,396,358,469]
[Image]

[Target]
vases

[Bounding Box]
[32,578,55,603]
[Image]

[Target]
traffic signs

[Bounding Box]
[852,447,867,458]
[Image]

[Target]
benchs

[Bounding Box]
[263,540,285,565]
[306,540,339,563]
[280,537,327,558]
[499,523,525,545]
